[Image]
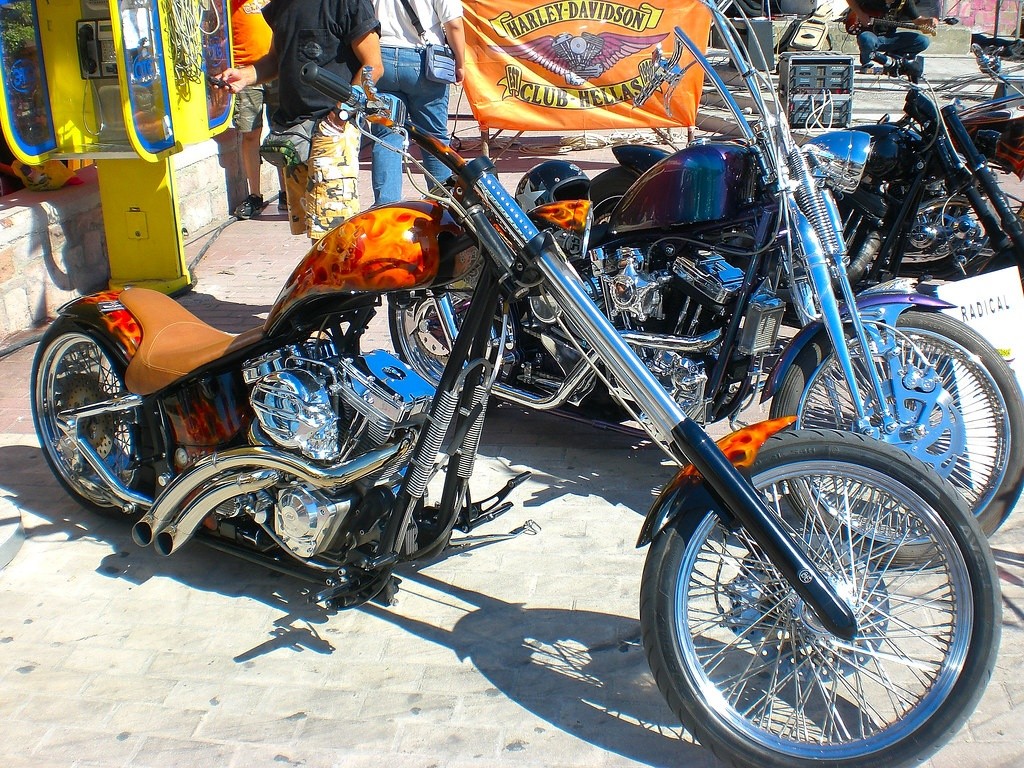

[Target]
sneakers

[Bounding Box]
[235,194,263,219]
[278,190,287,209]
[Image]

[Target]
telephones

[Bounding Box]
[74,17,118,80]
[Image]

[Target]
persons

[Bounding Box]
[210,0,384,248]
[0,36,47,190]
[369,0,467,207]
[847,0,941,75]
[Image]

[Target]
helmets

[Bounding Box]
[514,160,590,213]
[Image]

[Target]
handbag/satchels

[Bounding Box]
[259,118,315,178]
[12,159,85,192]
[425,44,457,84]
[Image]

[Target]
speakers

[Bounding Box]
[728,20,774,71]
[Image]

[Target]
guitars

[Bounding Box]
[844,6,937,36]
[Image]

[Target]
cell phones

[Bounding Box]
[207,75,232,90]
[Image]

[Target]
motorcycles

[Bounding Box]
[370,0,1022,570]
[28,60,1004,768]
